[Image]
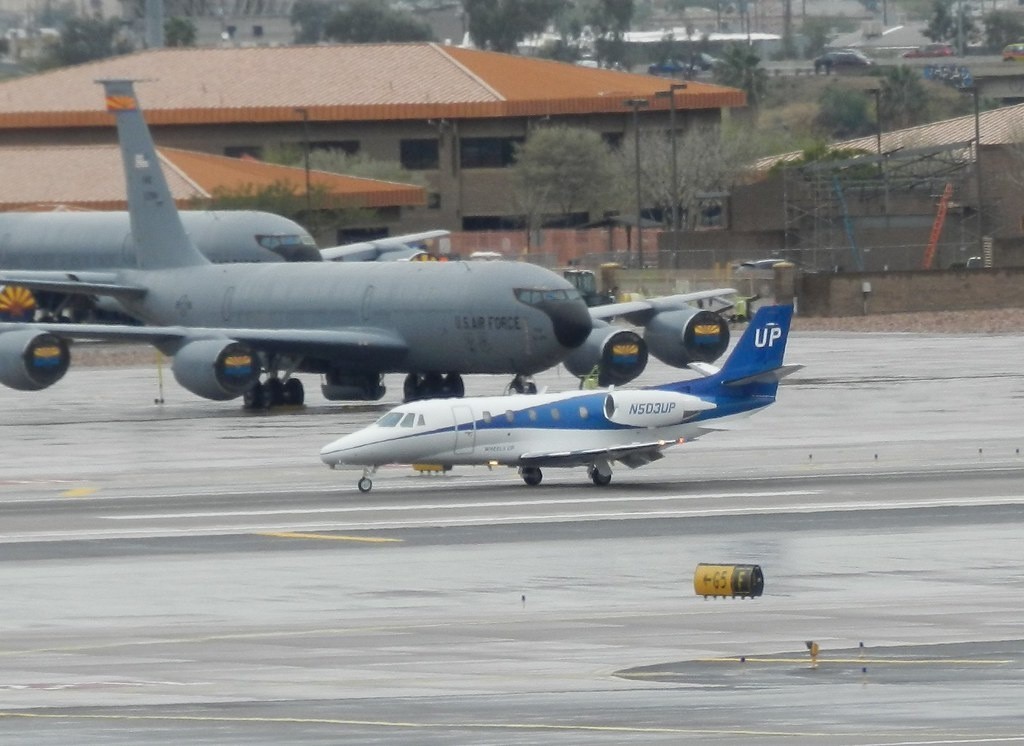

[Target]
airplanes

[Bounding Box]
[0,76,736,412]
[0,72,454,336]
[319,302,808,494]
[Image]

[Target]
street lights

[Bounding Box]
[862,88,882,220]
[958,76,985,274]
[624,98,650,270]
[654,84,688,269]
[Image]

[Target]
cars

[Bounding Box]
[695,53,724,72]
[813,49,875,75]
[1002,43,1024,61]
[901,41,952,59]
[648,58,701,78]
